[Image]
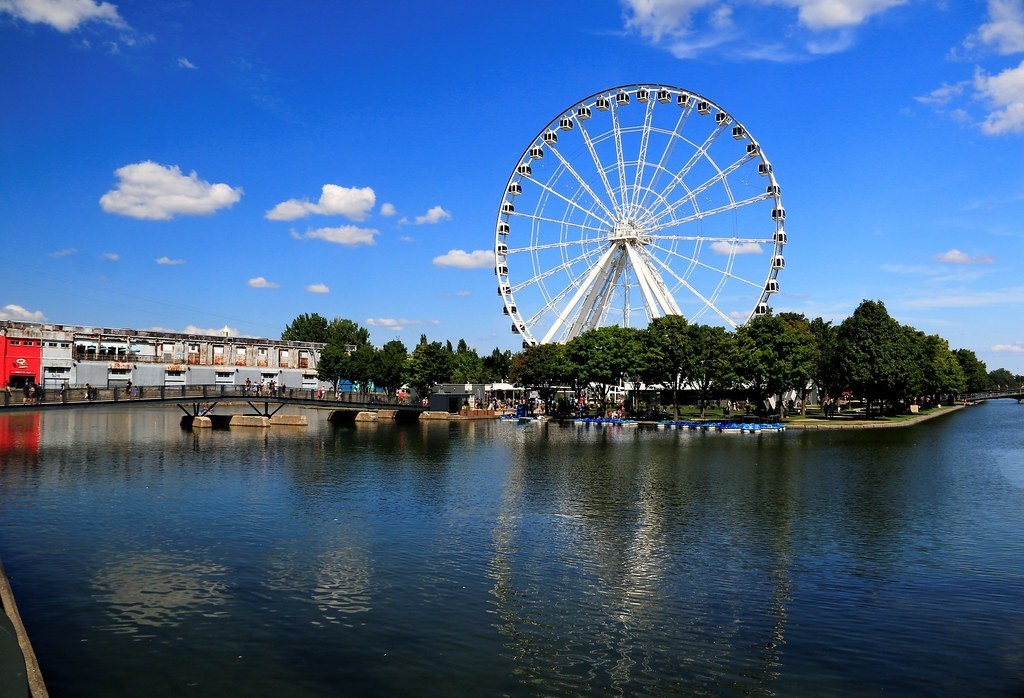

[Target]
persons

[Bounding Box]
[4,382,12,404]
[60,383,65,403]
[127,379,138,398]
[422,397,428,406]
[22,381,43,404]
[475,384,1024,419]
[399,389,405,404]
[245,377,286,398]
[320,385,343,401]
[86,383,91,400]
